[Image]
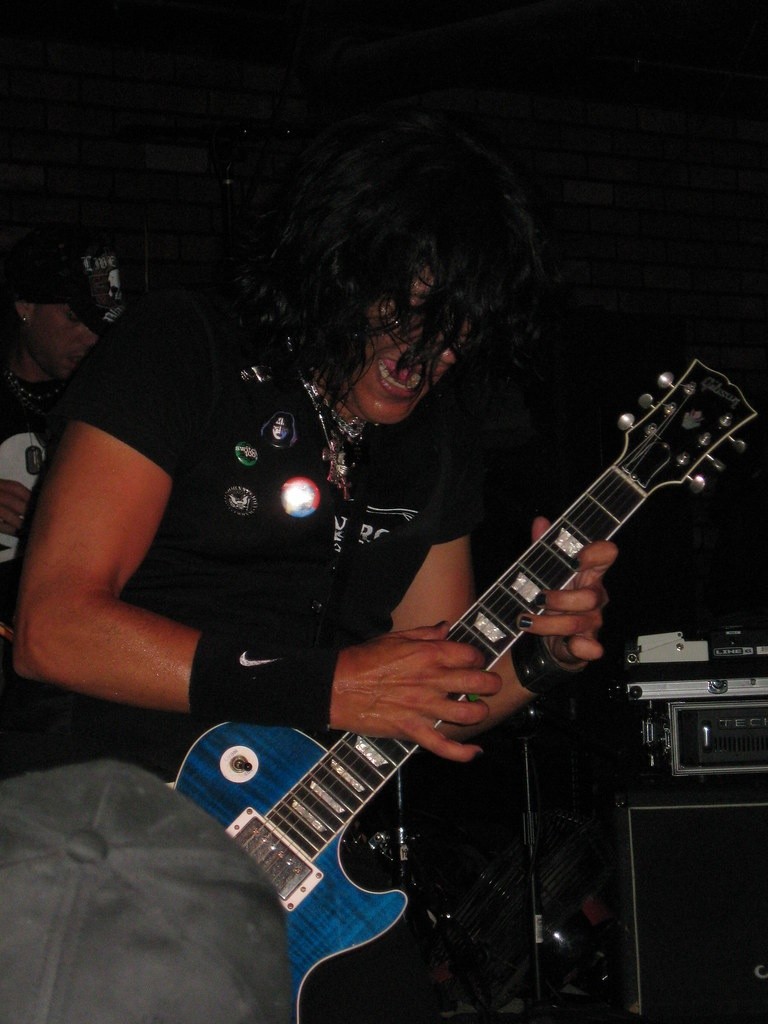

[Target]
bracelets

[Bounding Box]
[511,632,557,693]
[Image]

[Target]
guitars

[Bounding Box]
[165,354,756,1022]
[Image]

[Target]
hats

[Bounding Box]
[0,222,128,336]
[0,759,293,1024]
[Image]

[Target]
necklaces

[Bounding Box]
[4,366,65,415]
[286,335,363,500]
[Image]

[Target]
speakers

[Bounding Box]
[614,792,768,1024]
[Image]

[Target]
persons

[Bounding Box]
[0,225,167,773]
[12,101,617,1024]
